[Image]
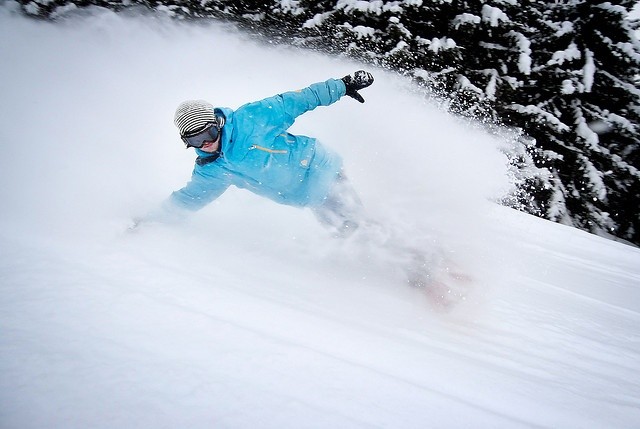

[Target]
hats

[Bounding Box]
[173,99,216,137]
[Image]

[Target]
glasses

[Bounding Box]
[181,125,219,149]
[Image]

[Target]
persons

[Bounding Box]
[122,70,433,291]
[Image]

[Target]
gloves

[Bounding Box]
[341,70,374,104]
